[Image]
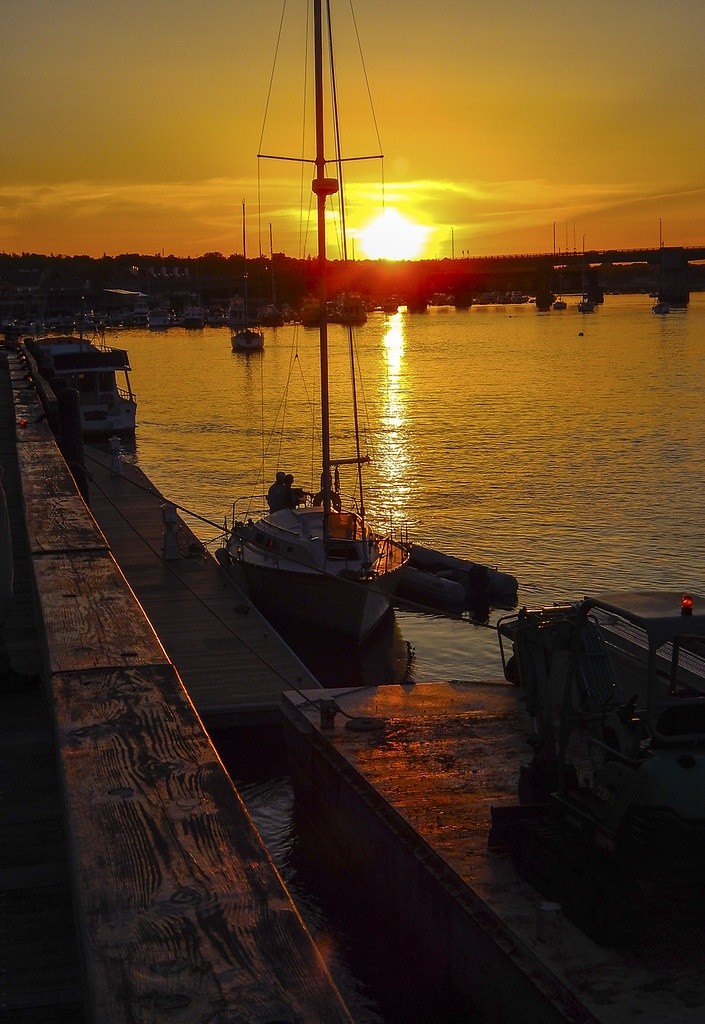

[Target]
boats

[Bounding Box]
[499,585,704,732]
[144,223,530,331]
[25,313,139,441]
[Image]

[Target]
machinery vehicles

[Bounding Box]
[488,694,705,952]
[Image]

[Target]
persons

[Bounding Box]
[267,472,300,514]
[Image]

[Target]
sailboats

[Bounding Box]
[577,255,597,313]
[229,203,266,352]
[217,0,411,644]
[651,249,672,315]
[552,269,567,309]
